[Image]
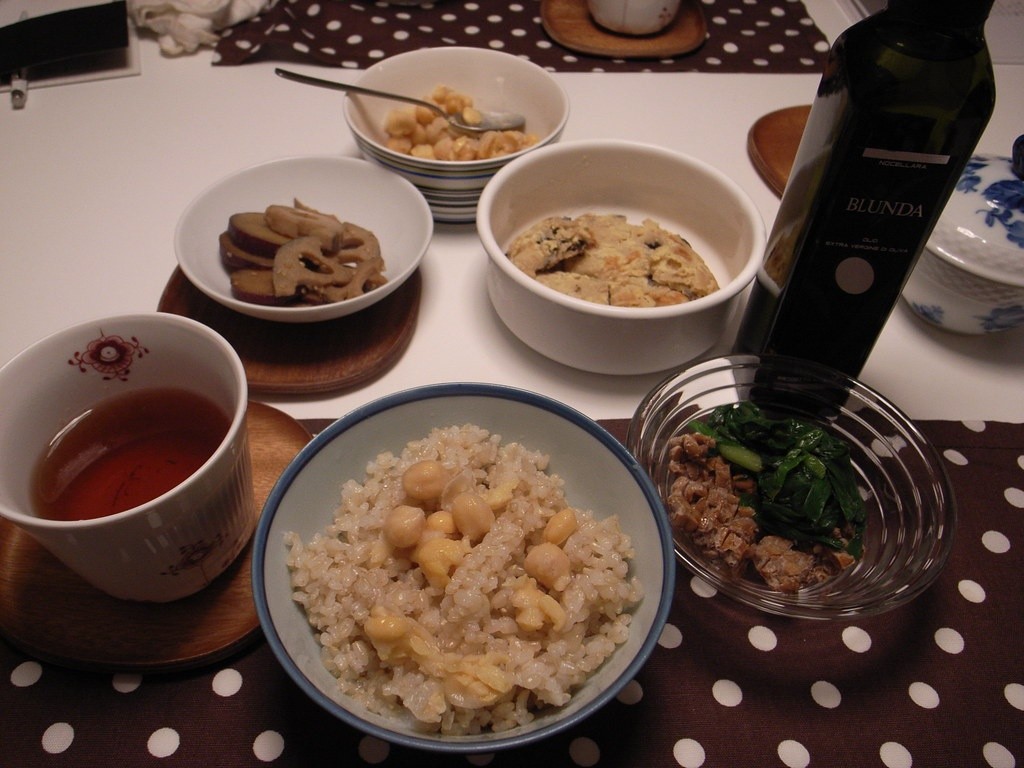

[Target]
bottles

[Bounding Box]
[730,0,997,420]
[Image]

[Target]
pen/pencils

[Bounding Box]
[10,9,29,109]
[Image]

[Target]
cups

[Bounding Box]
[0,312,255,606]
[583,0,682,39]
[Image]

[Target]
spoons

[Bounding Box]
[272,65,526,132]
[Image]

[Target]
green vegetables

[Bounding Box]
[687,400,867,560]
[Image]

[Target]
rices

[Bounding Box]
[282,422,644,737]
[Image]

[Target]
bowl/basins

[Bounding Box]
[172,153,432,324]
[624,352,957,630]
[476,139,767,379]
[255,381,677,753]
[901,135,1024,336]
[342,47,571,226]
